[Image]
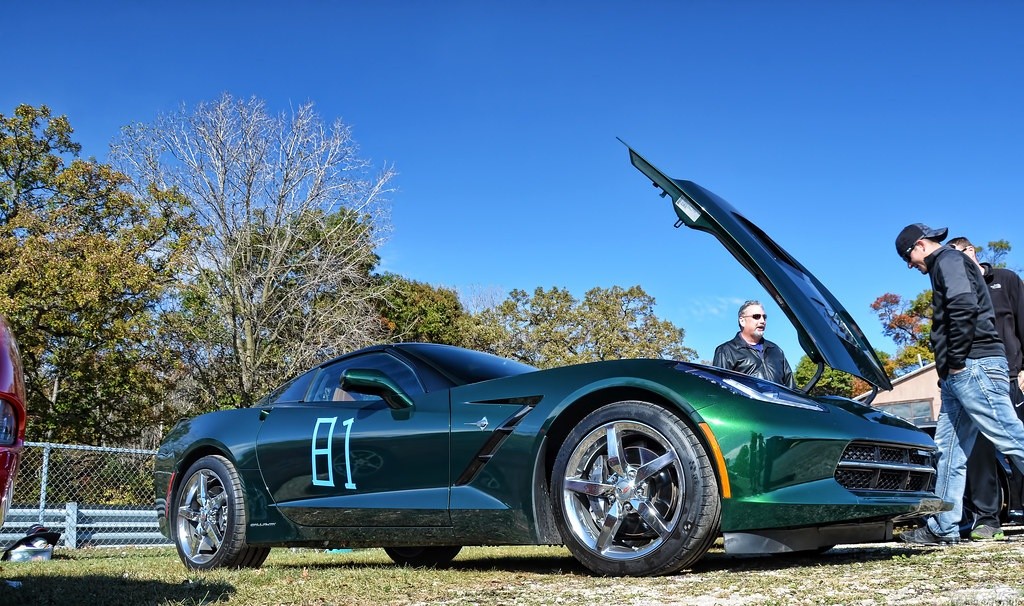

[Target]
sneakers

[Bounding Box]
[970,524,1004,539]
[900,524,960,543]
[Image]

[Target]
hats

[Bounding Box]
[895,223,948,257]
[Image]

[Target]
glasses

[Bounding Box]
[742,314,767,319]
[903,243,917,263]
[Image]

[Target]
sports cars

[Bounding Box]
[151,134,955,578]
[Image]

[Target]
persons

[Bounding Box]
[895,223,1024,547]
[946,237,1024,539]
[713,298,796,389]
